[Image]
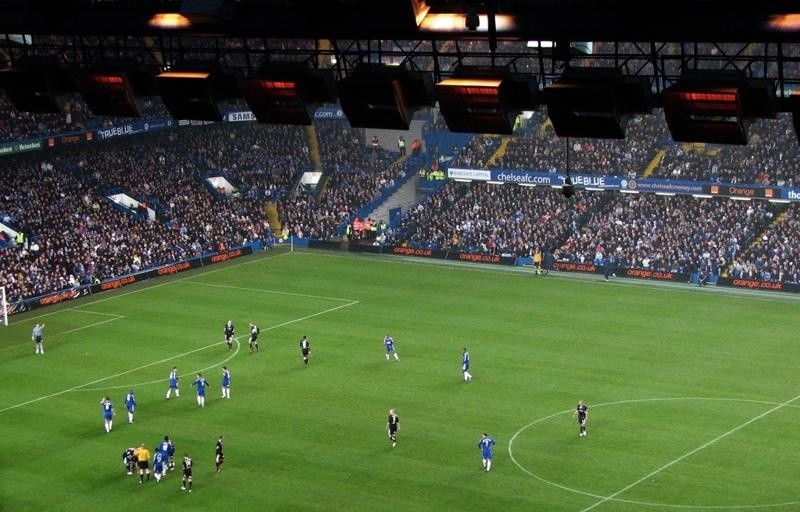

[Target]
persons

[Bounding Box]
[166,366,180,401]
[574,399,588,437]
[225,319,235,350]
[215,436,224,473]
[32,322,45,355]
[299,335,310,368]
[119,433,193,493]
[479,432,495,472]
[384,334,400,362]
[124,389,137,424]
[462,347,472,383]
[190,373,209,407]
[100,396,116,433]
[248,322,259,352]
[386,408,400,447]
[220,366,231,399]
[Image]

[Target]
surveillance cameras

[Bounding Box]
[562,184,574,198]
